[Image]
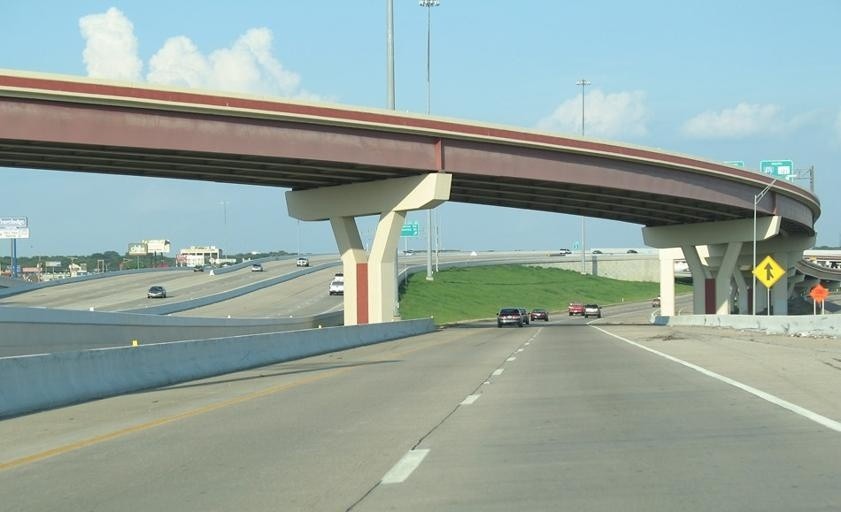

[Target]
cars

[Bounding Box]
[496,307,548,327]
[297,257,309,266]
[626,250,637,254]
[592,250,602,254]
[567,303,602,318]
[148,286,166,298]
[251,263,263,272]
[193,265,204,272]
[328,272,344,296]
[651,299,660,307]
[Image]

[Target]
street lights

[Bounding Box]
[419,0,440,282]
[576,79,592,275]
[752,174,797,315]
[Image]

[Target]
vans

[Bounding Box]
[558,248,572,255]
